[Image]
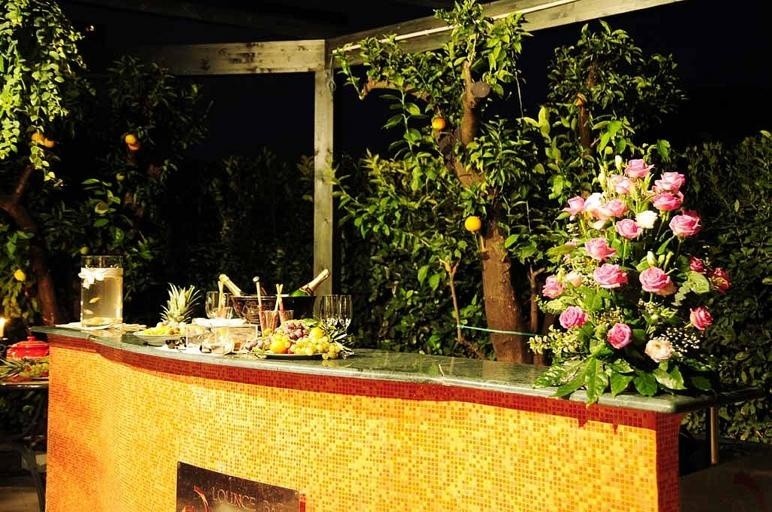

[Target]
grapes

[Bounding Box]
[268,321,340,359]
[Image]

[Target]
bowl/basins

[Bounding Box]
[229,294,317,324]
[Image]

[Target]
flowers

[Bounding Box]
[524,156,735,409]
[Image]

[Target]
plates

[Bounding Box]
[132,330,211,346]
[260,348,328,358]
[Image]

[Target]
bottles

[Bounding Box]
[219,273,250,297]
[289,268,331,297]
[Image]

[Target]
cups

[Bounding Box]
[205,291,234,319]
[319,294,340,327]
[80,255,124,329]
[334,294,354,334]
